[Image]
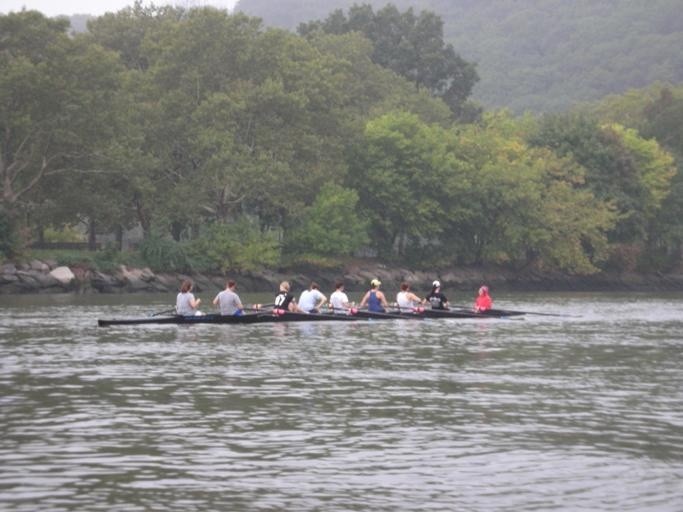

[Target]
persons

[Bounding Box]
[327,280,359,317]
[358,279,393,313]
[470,285,492,314]
[212,279,247,316]
[176,279,207,316]
[273,281,309,312]
[421,280,451,310]
[297,281,334,314]
[396,282,423,314]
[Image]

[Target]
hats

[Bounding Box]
[371,279,381,287]
[433,280,441,287]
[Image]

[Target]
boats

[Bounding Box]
[98,307,526,325]
